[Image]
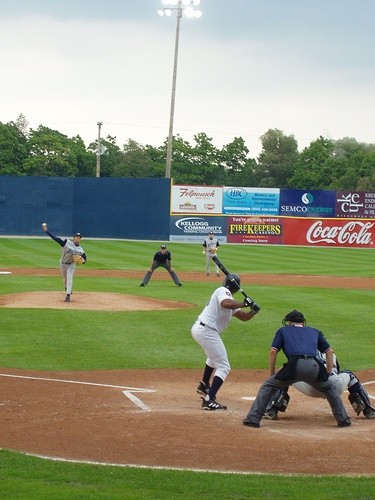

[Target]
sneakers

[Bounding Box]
[196,380,216,400]
[201,397,227,410]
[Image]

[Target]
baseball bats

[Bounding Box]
[212,256,260,312]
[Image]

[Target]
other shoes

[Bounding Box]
[262,409,279,419]
[242,419,260,428]
[338,417,351,427]
[216,273,220,277]
[363,405,375,419]
[178,282,182,287]
[139,283,145,287]
[64,294,70,302]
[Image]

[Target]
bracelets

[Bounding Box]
[216,248,217,250]
[203,251,205,253]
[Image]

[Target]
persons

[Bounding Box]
[202,231,220,277]
[42,225,87,302]
[261,349,375,420]
[139,244,183,287]
[191,273,260,410]
[243,310,351,428]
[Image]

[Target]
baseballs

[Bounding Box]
[42,223,46,228]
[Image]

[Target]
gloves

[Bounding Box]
[243,296,253,307]
[250,303,260,314]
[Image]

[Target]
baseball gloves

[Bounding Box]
[73,254,85,264]
[211,247,217,254]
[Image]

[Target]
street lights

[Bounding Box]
[158,0,202,177]
[97,121,102,177]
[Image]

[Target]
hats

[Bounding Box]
[161,244,167,249]
[73,233,82,238]
[208,231,214,235]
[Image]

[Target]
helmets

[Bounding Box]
[225,274,240,292]
[284,310,304,323]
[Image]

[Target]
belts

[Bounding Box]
[62,262,71,265]
